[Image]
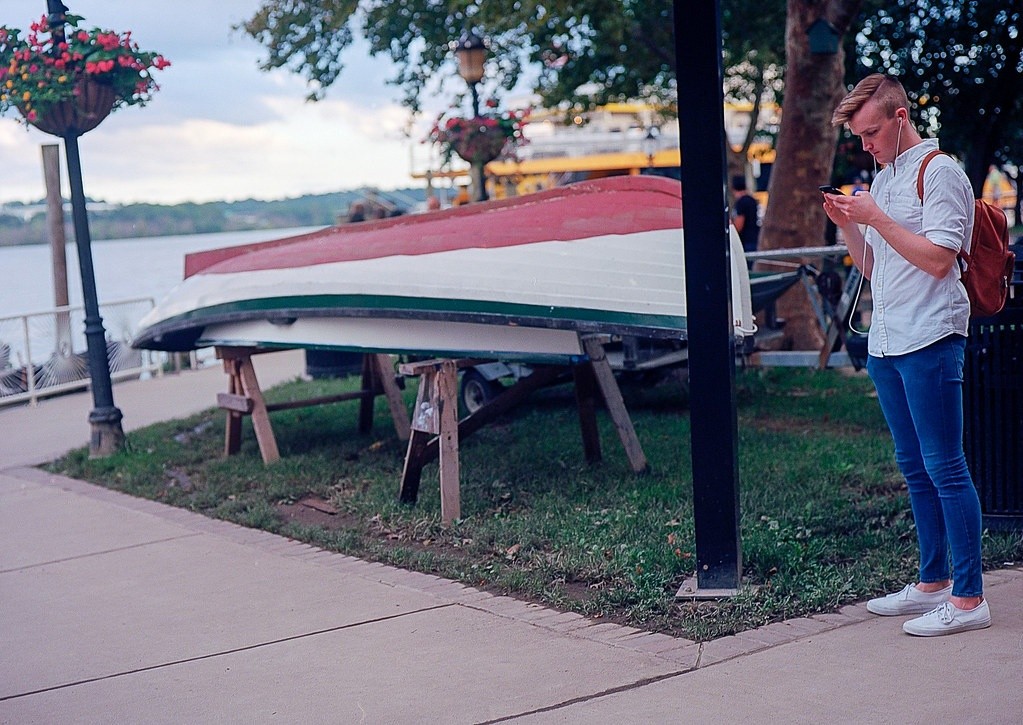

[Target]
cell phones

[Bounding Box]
[819,185,844,195]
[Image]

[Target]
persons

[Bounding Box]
[732,176,758,270]
[822,73,991,636]
[344,199,404,225]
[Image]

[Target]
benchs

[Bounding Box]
[215,346,413,466]
[396,333,652,529]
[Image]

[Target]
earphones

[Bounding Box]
[898,116,902,122]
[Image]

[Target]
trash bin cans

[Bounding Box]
[305,350,365,378]
[961,270,1023,532]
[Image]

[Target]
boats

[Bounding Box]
[127,177,756,376]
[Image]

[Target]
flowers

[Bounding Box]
[0,14,171,131]
[420,96,539,172]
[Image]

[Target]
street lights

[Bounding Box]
[456,30,498,203]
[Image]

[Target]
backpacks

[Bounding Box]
[918,150,1014,317]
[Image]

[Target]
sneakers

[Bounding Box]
[866,583,953,614]
[903,599,992,636]
